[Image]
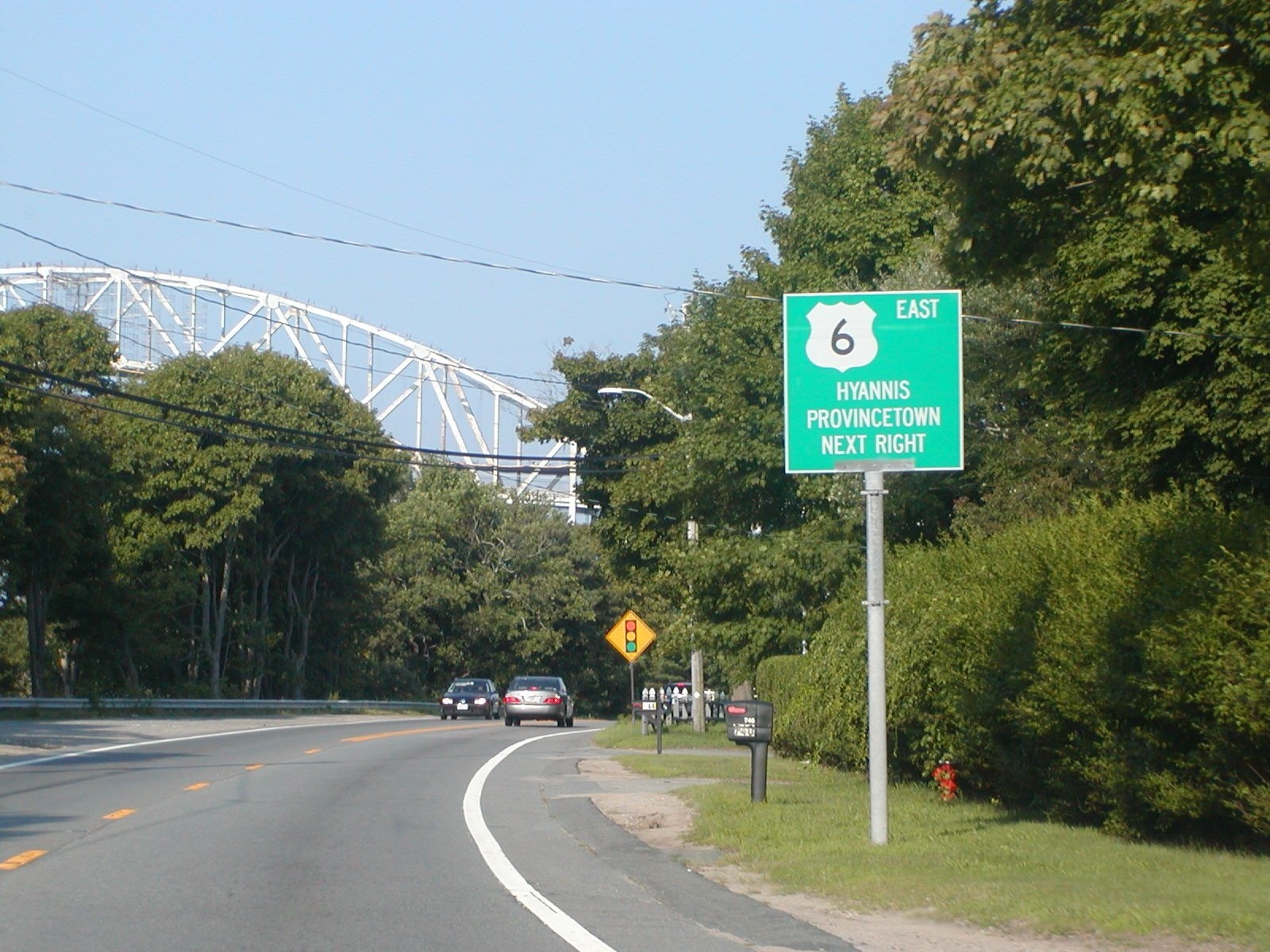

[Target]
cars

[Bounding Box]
[662,682,692,716]
[439,677,502,721]
[503,675,575,728]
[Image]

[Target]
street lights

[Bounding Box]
[594,386,705,731]
